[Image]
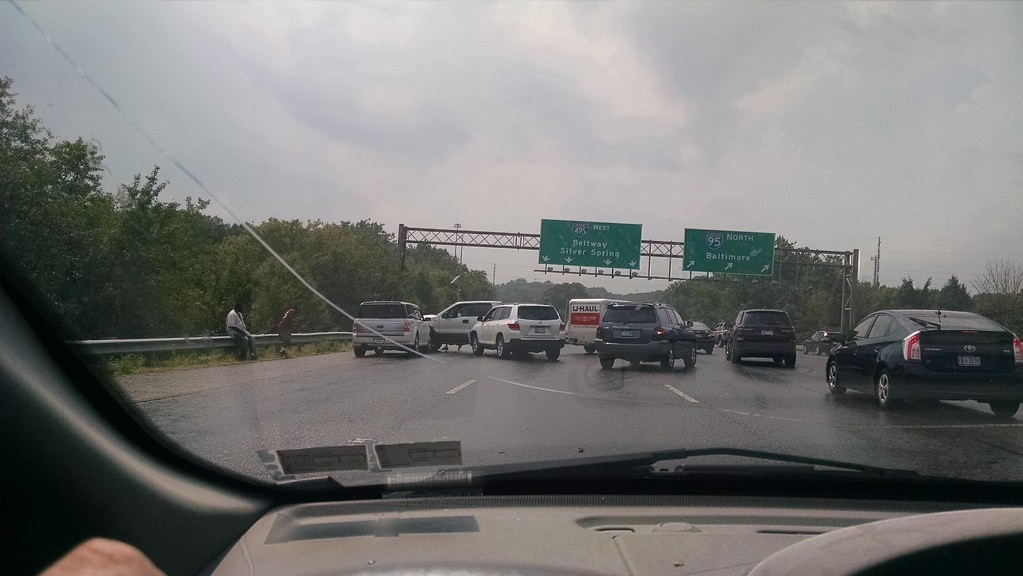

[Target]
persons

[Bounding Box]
[226,303,258,361]
[277,305,298,358]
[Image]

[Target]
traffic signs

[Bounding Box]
[538,219,642,270]
[682,228,775,276]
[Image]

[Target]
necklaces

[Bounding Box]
[237,313,242,320]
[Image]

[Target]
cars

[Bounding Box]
[685,321,715,354]
[725,309,797,369]
[352,301,430,357]
[803,331,841,355]
[468,303,566,361]
[826,310,1023,418]
[712,323,734,348]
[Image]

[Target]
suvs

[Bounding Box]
[594,301,697,370]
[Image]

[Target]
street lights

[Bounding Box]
[454,224,461,257]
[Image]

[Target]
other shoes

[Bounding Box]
[238,355,248,360]
[249,355,258,360]
[280,350,287,355]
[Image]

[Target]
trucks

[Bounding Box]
[568,299,630,353]
[427,301,503,350]
